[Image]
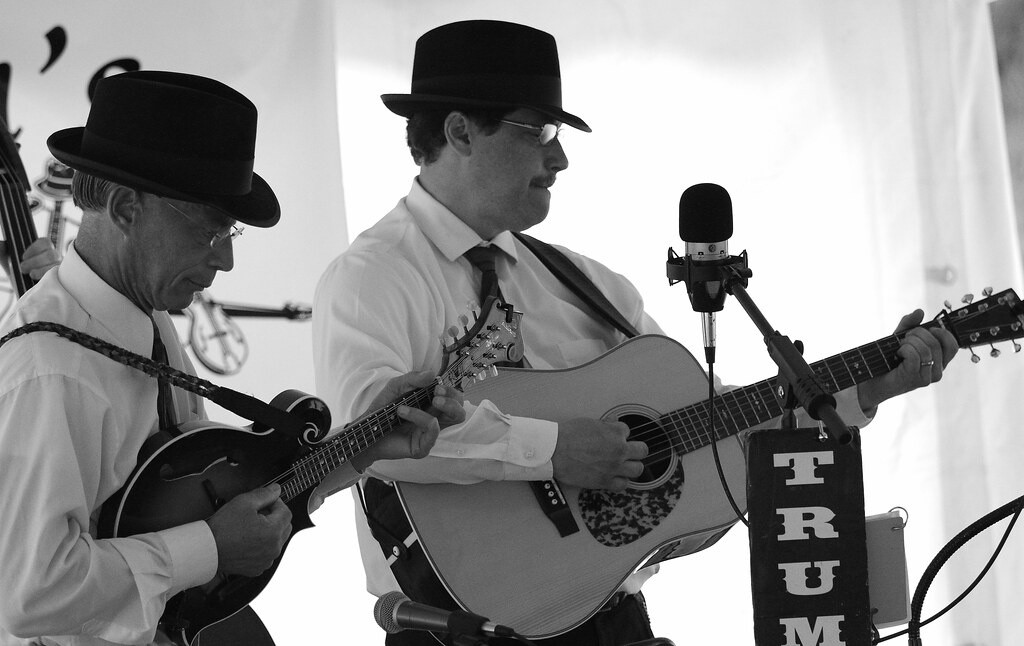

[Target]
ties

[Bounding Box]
[464,248,533,369]
[150,330,181,429]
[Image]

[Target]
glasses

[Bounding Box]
[157,196,244,249]
[471,113,568,145]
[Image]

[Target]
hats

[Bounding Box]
[46,70,281,228]
[380,20,591,133]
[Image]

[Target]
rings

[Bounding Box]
[921,361,934,366]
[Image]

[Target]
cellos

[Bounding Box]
[0,59,276,644]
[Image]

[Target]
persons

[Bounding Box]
[311,18,960,646]
[0,71,465,646]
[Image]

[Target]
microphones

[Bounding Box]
[679,183,734,364]
[374,590,514,638]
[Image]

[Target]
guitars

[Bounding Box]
[361,281,1024,645]
[95,294,528,646]
